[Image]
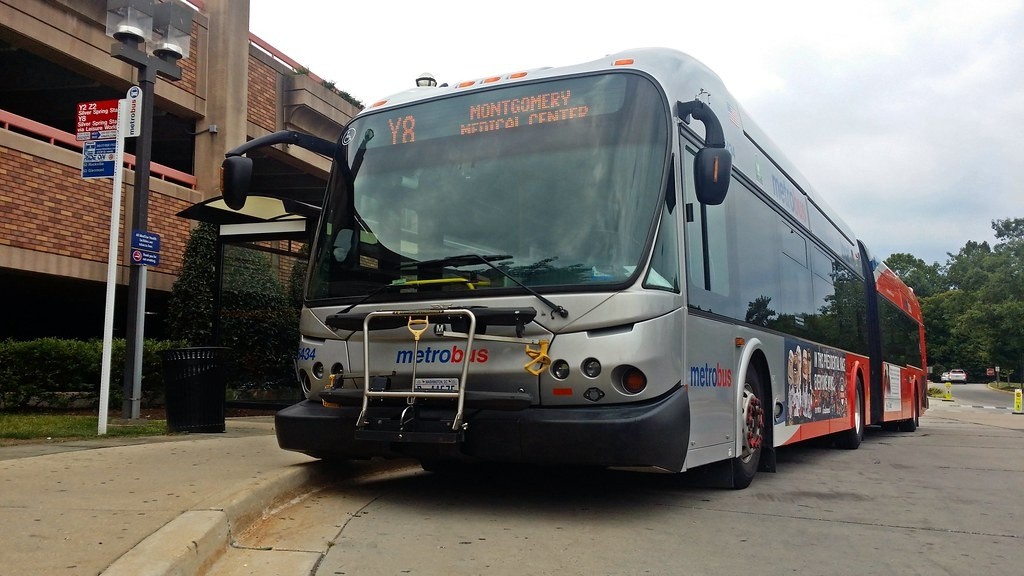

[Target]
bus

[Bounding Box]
[218,44,929,492]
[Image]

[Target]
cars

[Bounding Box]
[947,368,968,384]
[940,371,953,383]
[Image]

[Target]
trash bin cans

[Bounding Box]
[153,345,233,433]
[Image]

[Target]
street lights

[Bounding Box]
[108,22,183,418]
